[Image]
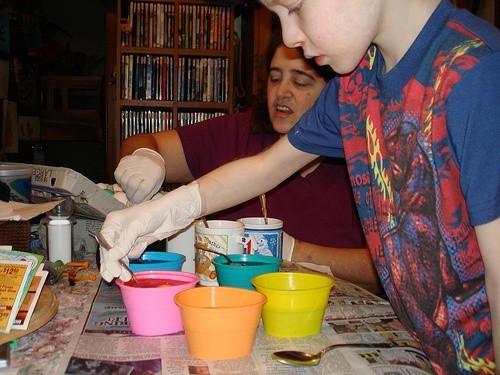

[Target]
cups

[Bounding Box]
[196,219,245,287]
[240,217,285,257]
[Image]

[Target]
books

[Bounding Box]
[119,1,233,146]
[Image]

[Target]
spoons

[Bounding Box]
[272,341,393,367]
[195,244,246,266]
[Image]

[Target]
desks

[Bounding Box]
[0,244,435,375]
[32,141,105,185]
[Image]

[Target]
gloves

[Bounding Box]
[100,181,202,282]
[282,231,295,262]
[114,148,165,205]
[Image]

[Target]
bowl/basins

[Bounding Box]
[115,270,200,335]
[174,287,267,359]
[251,272,337,338]
[128,251,185,272]
[211,254,284,291]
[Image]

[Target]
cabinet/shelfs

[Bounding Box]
[105,0,234,184]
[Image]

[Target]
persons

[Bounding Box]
[96,0,500,375]
[113,29,383,295]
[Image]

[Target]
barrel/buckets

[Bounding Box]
[3,165,34,207]
